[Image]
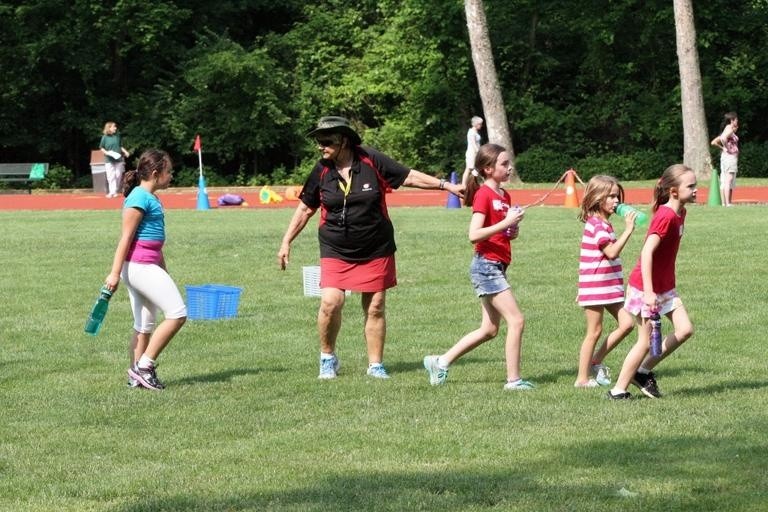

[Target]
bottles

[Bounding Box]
[84,284,112,336]
[505,205,519,238]
[614,203,648,228]
[649,313,663,357]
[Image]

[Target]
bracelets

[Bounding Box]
[440,176,448,192]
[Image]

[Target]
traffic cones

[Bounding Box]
[446,171,463,208]
[563,171,580,208]
[196,175,211,209]
[705,168,724,208]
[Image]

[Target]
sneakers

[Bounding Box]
[631,371,661,398]
[128,377,140,387]
[574,378,599,388]
[367,364,390,378]
[591,361,612,384]
[503,379,535,390]
[607,390,631,399]
[424,355,448,385]
[128,361,165,390]
[318,353,340,379]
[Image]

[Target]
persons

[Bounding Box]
[605,163,699,402]
[99,122,130,198]
[277,115,467,381]
[572,176,638,389]
[462,115,487,189]
[423,143,534,392]
[105,148,188,389]
[710,111,741,207]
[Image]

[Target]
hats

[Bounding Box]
[306,116,361,146]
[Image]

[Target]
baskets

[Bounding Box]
[302,266,351,297]
[184,283,244,320]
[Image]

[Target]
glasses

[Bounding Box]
[316,138,334,146]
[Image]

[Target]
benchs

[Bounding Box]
[0,162,49,195]
